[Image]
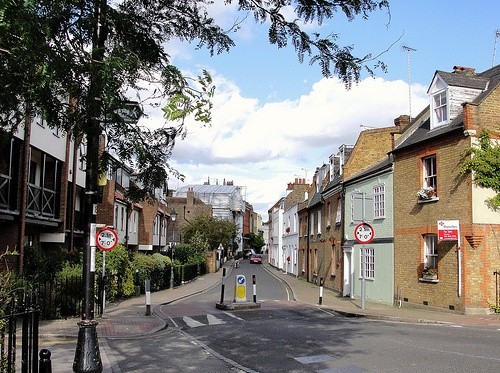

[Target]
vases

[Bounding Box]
[422,272,437,280]
[418,191,433,200]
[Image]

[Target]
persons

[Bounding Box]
[227,254,234,267]
[249,251,251,258]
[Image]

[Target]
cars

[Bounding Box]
[250,254,263,264]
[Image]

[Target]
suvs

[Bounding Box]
[243,249,255,260]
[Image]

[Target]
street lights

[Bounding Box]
[170,208,177,291]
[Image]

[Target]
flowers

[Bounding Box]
[416,266,437,275]
[417,186,434,198]
